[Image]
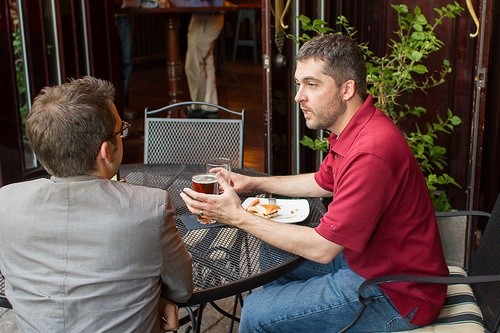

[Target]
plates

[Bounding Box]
[242,197,310,223]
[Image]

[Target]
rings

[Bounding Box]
[200,209,203,216]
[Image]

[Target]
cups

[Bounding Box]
[191,174,219,225]
[205,158,231,194]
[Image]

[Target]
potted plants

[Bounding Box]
[286,1,467,268]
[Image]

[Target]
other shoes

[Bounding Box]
[182,109,220,119]
[122,107,137,118]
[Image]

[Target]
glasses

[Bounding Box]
[111,120,132,137]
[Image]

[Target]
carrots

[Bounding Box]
[250,199,259,206]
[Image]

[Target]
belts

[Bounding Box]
[193,11,222,15]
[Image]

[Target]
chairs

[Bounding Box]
[333,195,500,333]
[144,101,252,293]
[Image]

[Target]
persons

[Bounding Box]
[180,34,450,333]
[0,76,193,333]
[117,0,225,117]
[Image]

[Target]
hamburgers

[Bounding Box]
[248,204,280,218]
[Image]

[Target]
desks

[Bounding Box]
[128,6,239,120]
[120,163,326,333]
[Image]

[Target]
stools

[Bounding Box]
[233,4,260,64]
